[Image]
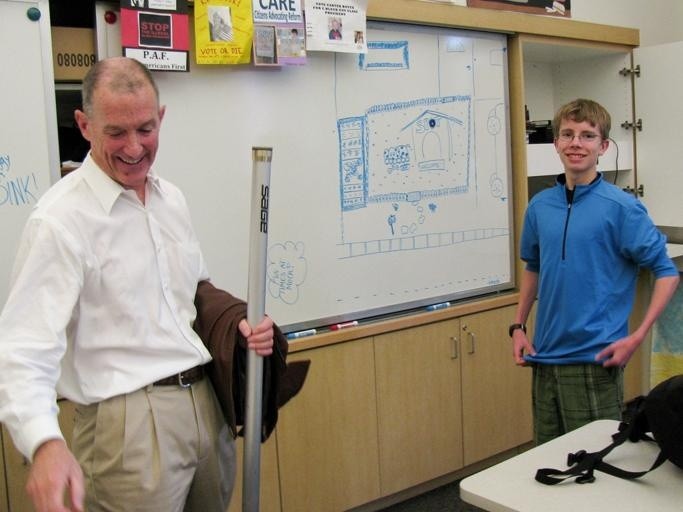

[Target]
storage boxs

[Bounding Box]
[51,25,96,82]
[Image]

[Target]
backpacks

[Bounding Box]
[538,372,683,485]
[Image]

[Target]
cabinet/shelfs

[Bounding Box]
[228,335,380,512]
[375,304,537,512]
[507,33,683,292]
[1,401,76,511]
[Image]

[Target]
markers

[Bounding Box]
[424,302,451,311]
[287,329,316,339]
[329,320,359,331]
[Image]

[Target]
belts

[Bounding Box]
[150,366,211,388]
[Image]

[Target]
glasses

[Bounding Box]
[558,134,603,142]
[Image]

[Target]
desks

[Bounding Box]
[458,419,683,512]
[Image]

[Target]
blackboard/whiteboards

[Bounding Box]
[95,1,516,336]
[0,0,62,315]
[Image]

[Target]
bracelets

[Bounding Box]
[509,322,527,337]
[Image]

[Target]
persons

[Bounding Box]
[354,31,365,44]
[328,20,342,40]
[507,98,682,448]
[0,57,276,512]
[287,28,301,54]
